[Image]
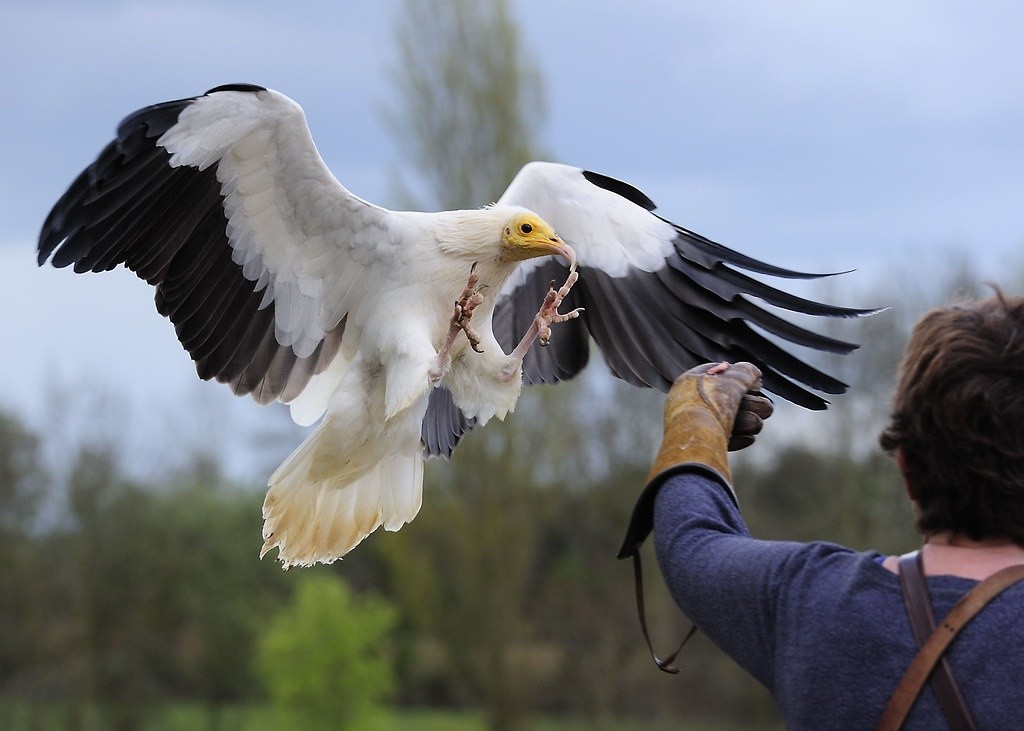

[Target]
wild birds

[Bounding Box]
[38,83,893,570]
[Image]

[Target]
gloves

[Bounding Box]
[617,361,774,560]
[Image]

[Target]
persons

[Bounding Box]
[649,281,1024,731]
